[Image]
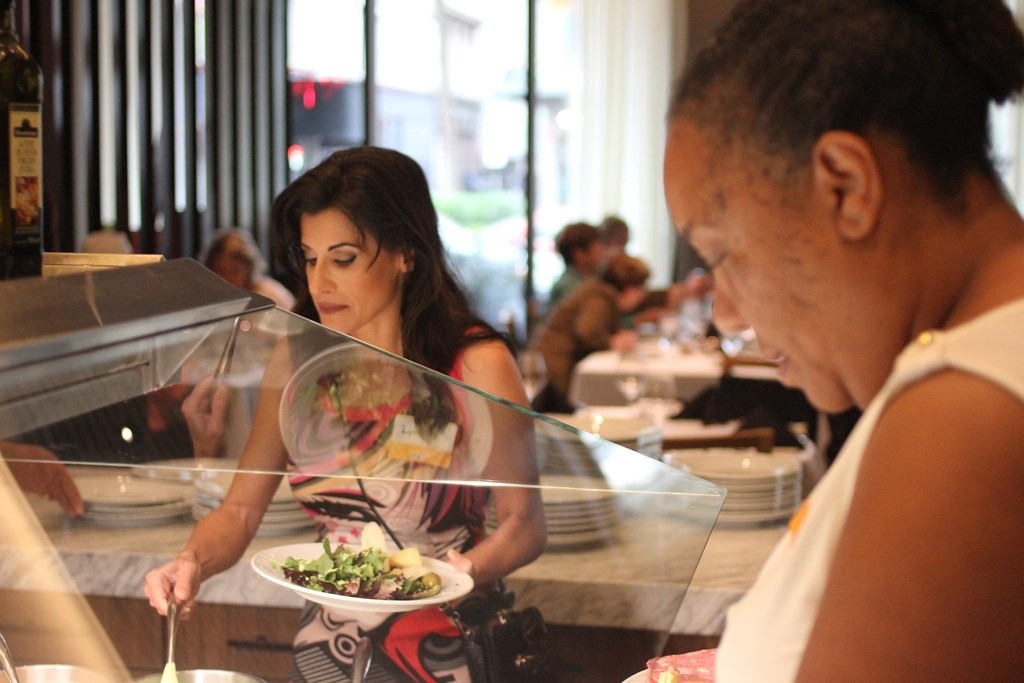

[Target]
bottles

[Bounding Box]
[0,1,42,279]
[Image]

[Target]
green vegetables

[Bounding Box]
[266,536,427,599]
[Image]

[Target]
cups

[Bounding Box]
[516,349,549,403]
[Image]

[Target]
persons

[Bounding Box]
[142,147,548,683]
[202,230,298,338]
[663,0,1024,683]
[181,375,230,468]
[0,438,85,519]
[518,216,659,415]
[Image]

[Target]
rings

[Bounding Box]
[41,493,50,503]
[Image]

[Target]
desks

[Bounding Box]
[571,352,829,447]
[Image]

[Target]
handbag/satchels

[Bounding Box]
[466,607,556,683]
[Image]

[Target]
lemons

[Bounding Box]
[389,546,423,566]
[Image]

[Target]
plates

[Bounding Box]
[72,456,320,537]
[250,541,474,613]
[479,406,807,548]
